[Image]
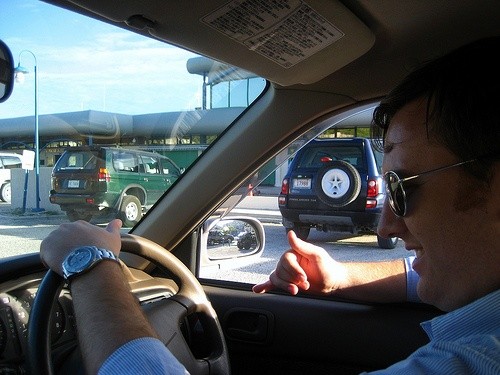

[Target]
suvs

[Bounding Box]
[48,142,187,228]
[277,135,399,250]
[236,232,257,250]
[207,230,235,246]
[0,153,24,202]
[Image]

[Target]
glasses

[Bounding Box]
[382,155,475,218]
